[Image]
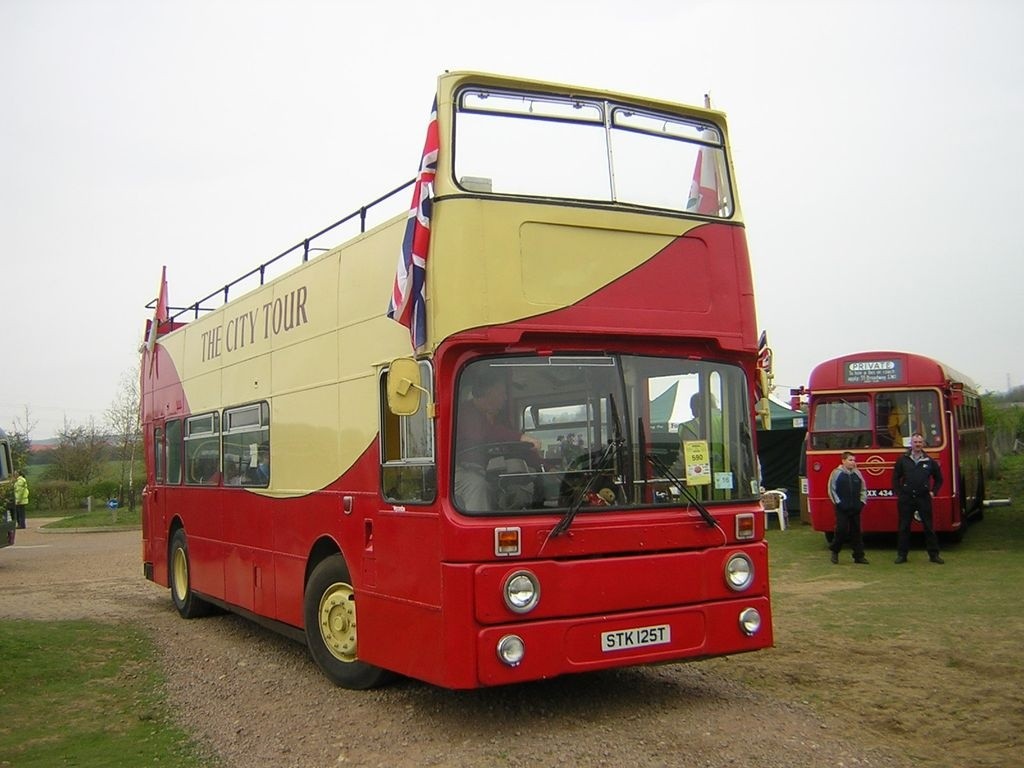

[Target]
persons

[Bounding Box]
[555,433,584,468]
[654,391,762,503]
[208,441,270,485]
[823,410,861,449]
[893,433,944,564]
[453,366,522,512]
[106,496,119,524]
[827,452,870,564]
[888,392,927,447]
[14,470,29,528]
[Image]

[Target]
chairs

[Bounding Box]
[760,490,787,531]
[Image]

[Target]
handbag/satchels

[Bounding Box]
[898,417,917,435]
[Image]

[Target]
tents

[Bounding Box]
[755,398,808,432]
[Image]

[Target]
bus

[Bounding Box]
[139,69,776,691]
[790,351,987,544]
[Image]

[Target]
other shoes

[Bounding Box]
[16,526,25,529]
[831,550,838,563]
[895,555,906,563]
[930,555,944,563]
[854,557,869,564]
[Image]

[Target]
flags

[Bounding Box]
[386,93,437,349]
[686,96,720,217]
[156,266,167,323]
[753,331,771,416]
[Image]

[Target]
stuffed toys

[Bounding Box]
[586,485,619,506]
